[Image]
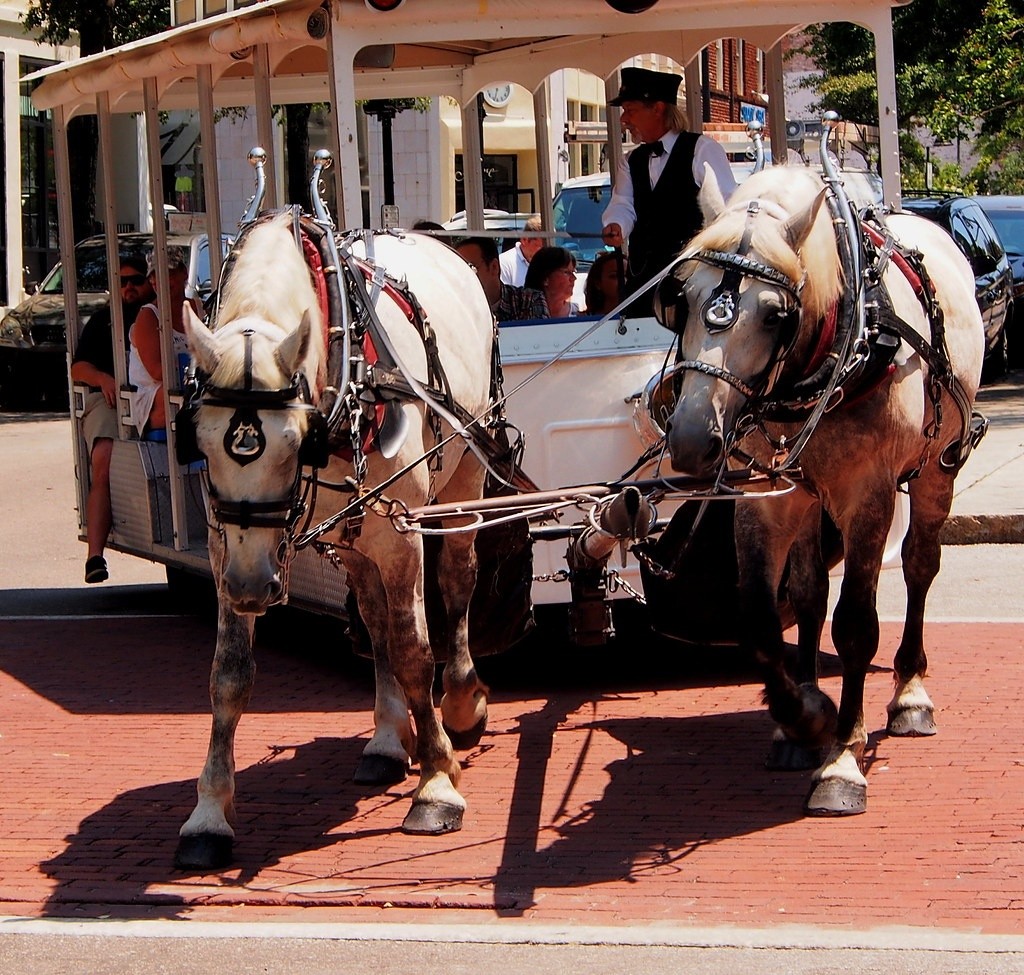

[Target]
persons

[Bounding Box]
[576,251,629,317]
[454,237,550,321]
[128,245,208,440]
[69,255,157,583]
[524,247,583,318]
[601,66,738,317]
[411,221,452,246]
[497,216,557,287]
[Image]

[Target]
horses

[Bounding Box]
[665,164,985,816]
[177,205,493,868]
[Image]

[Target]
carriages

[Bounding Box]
[15,1,992,868]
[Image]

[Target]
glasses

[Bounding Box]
[557,268,576,276]
[120,273,147,288]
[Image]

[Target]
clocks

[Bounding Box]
[482,83,514,108]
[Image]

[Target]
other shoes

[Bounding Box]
[85,556,109,584]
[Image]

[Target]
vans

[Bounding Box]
[539,160,884,316]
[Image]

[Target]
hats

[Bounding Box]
[144,246,185,278]
[606,67,683,109]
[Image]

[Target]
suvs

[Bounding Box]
[872,189,1016,386]
[0,229,240,414]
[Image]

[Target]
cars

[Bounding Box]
[437,207,539,260]
[960,195,1024,367]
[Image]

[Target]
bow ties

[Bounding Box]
[645,140,664,156]
